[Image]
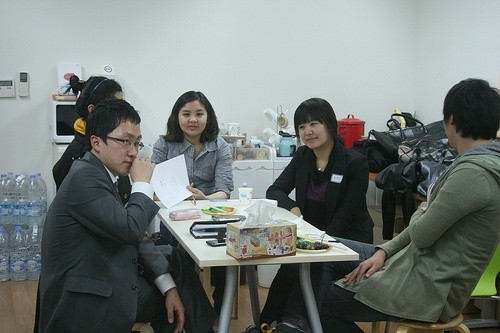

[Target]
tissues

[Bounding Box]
[225,199,298,261]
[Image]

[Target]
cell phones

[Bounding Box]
[206,239,227,247]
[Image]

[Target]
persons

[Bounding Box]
[245,78,500,333]
[245,98,375,333]
[32,99,219,333]
[150,90,233,316]
[53,74,197,275]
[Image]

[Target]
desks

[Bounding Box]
[155,201,360,333]
[370,170,426,205]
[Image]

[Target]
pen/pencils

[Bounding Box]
[212,239,227,243]
[193,193,196,205]
[210,205,227,214]
[322,238,340,243]
[202,228,226,232]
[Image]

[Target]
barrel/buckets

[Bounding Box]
[337,113,365,148]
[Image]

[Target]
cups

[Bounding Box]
[238,187,253,206]
[263,107,278,121]
[263,127,276,138]
[269,132,283,151]
[228,123,240,136]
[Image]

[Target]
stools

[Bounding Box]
[374,312,470,333]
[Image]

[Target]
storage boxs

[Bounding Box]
[236,144,270,159]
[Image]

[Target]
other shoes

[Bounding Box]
[212,288,222,316]
[272,323,300,333]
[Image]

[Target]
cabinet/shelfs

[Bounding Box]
[275,158,296,201]
[229,159,275,200]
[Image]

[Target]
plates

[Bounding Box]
[296,240,333,253]
[201,206,236,215]
[211,215,246,221]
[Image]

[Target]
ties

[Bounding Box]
[114,181,119,193]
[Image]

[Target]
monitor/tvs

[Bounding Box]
[52,101,76,143]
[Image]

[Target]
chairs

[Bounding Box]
[464,242,500,333]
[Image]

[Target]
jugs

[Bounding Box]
[279,137,296,157]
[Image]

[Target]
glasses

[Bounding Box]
[94,134,144,150]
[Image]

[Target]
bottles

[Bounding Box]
[0,172,49,283]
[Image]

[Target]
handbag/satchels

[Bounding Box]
[355,119,458,198]
